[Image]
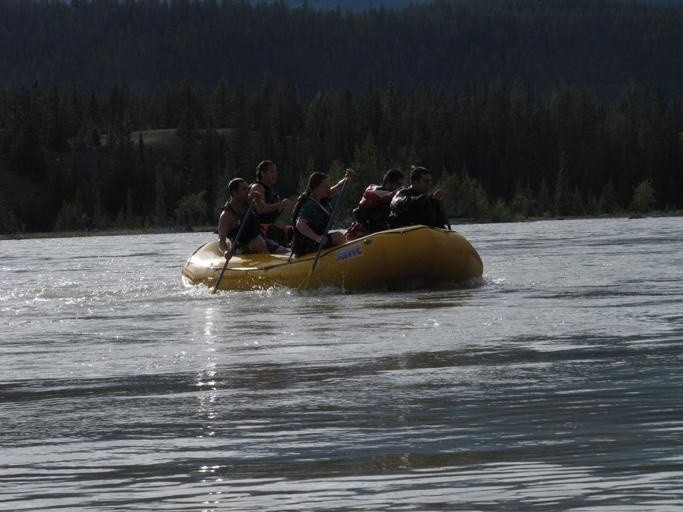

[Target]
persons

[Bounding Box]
[217,160,447,259]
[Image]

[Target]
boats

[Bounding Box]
[178,222,483,296]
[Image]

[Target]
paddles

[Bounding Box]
[297,178,347,293]
